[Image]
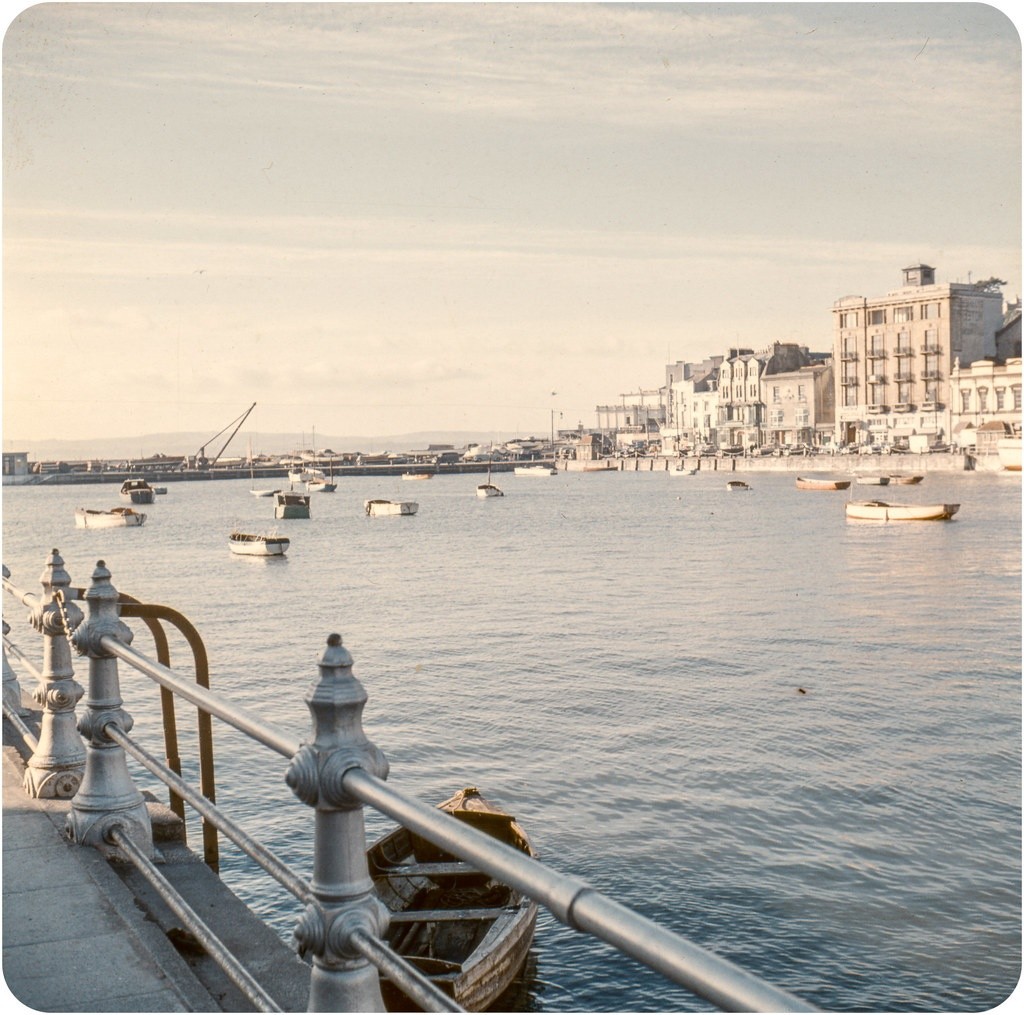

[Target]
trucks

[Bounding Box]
[474,447,503,462]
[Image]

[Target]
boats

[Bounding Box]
[845,498,960,520]
[155,486,167,494]
[402,472,433,481]
[856,476,890,485]
[364,499,419,515]
[795,476,851,490]
[727,479,749,491]
[273,491,310,519]
[670,465,695,475]
[119,478,155,504]
[75,506,148,526]
[583,467,618,472]
[513,465,557,476]
[228,532,290,555]
[365,786,539,1013]
[888,474,924,484]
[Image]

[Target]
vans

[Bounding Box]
[432,451,459,465]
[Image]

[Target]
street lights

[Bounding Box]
[551,410,563,451]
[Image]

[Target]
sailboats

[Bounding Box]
[477,440,504,497]
[288,431,325,482]
[248,436,281,495]
[306,425,337,492]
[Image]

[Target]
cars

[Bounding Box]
[679,442,951,456]
